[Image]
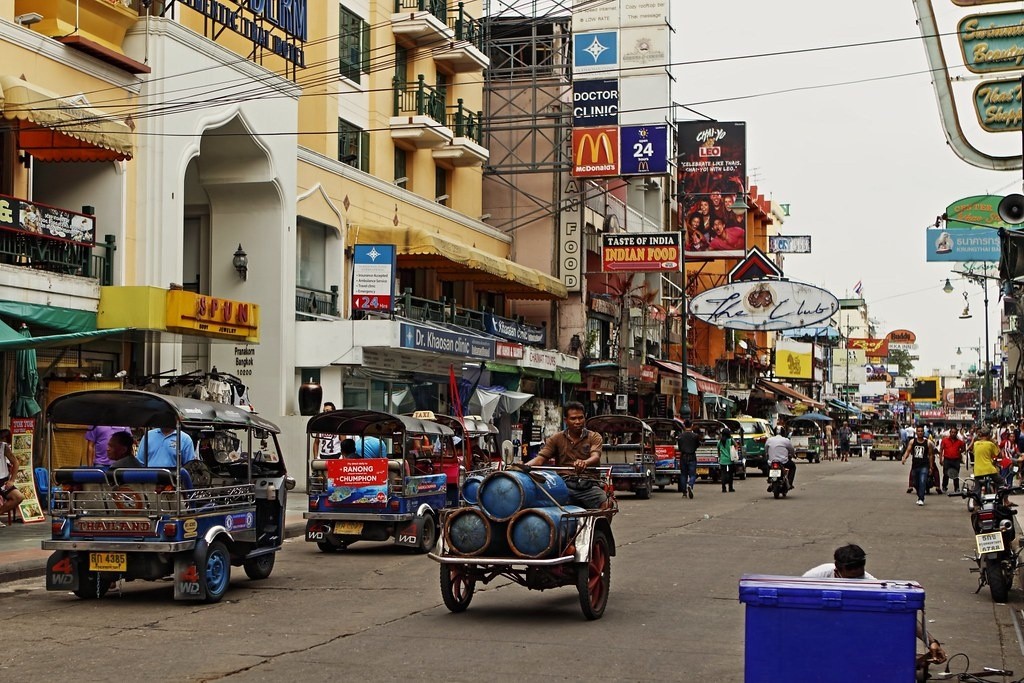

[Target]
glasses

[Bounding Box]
[841,558,866,571]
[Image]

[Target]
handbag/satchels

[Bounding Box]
[729,439,739,461]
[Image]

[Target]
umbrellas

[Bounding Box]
[9,323,41,419]
[794,412,834,421]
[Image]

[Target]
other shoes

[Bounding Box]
[687,486,693,499]
[722,488,727,493]
[1004,501,1019,508]
[906,488,913,493]
[935,488,942,494]
[917,500,924,506]
[682,494,687,498]
[729,488,735,492]
[916,499,919,505]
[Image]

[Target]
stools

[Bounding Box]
[0,498,16,526]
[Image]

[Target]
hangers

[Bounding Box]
[163,374,250,390]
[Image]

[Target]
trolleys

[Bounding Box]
[426,463,621,621]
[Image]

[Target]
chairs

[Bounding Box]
[35,468,62,511]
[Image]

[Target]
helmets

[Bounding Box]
[773,425,786,437]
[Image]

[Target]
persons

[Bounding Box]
[136,428,195,468]
[677,420,700,499]
[19,203,37,232]
[524,400,612,524]
[313,402,346,460]
[824,422,833,445]
[512,435,520,457]
[354,435,387,458]
[681,177,747,251]
[717,428,736,492]
[838,421,852,461]
[85,426,132,472]
[764,426,796,491]
[0,441,24,527]
[898,423,1024,505]
[802,544,947,664]
[340,439,362,459]
[106,431,141,470]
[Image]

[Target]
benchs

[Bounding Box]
[51,465,215,516]
[696,441,720,457]
[653,440,676,461]
[598,444,642,463]
[311,459,410,494]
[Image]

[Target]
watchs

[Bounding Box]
[930,639,940,646]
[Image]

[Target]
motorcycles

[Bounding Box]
[945,451,1024,603]
[640,416,688,492]
[584,414,657,500]
[835,429,908,461]
[684,417,776,483]
[783,417,825,464]
[303,408,515,553]
[40,387,297,604]
[766,458,790,499]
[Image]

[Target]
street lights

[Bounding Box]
[672,176,752,419]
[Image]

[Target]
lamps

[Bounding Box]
[435,194,449,203]
[393,177,409,186]
[19,150,31,168]
[479,213,491,222]
[344,154,357,164]
[232,244,248,282]
[344,245,352,259]
[14,12,44,29]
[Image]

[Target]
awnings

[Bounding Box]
[658,361,735,407]
[347,224,569,302]
[0,74,134,161]
[762,381,861,414]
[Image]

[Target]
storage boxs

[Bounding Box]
[738,575,925,683]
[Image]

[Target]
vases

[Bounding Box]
[298,382,323,416]
